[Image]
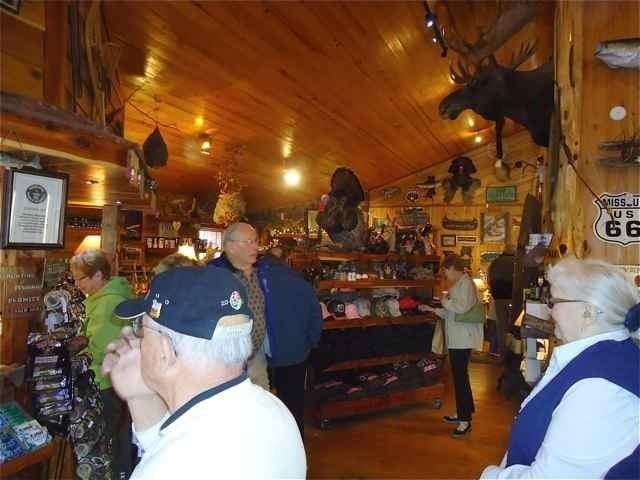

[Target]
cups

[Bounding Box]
[144,236,208,249]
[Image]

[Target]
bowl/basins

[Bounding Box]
[373,217,384,228]
[523,233,553,254]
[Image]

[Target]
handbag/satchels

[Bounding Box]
[454,303,484,323]
[431,320,449,355]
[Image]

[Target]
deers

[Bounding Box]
[439,37,554,147]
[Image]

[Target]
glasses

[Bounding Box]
[132,317,165,338]
[547,294,585,310]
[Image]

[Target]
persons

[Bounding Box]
[478,256,640,480]
[99,265,308,480]
[260,247,322,443]
[207,222,276,392]
[487,243,517,357]
[69,249,139,480]
[417,255,485,438]
[148,251,201,278]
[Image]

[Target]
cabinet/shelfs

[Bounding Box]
[289,246,447,429]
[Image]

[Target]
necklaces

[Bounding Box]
[159,371,248,433]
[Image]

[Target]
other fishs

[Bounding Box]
[593,38,640,71]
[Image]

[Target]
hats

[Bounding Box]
[113,267,254,339]
[319,295,418,320]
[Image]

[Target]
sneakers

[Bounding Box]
[453,421,472,438]
[442,416,458,423]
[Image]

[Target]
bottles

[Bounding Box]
[4,272,41,312]
[321,265,383,281]
[524,269,550,301]
[128,262,149,296]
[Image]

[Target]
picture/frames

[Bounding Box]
[2,165,69,249]
